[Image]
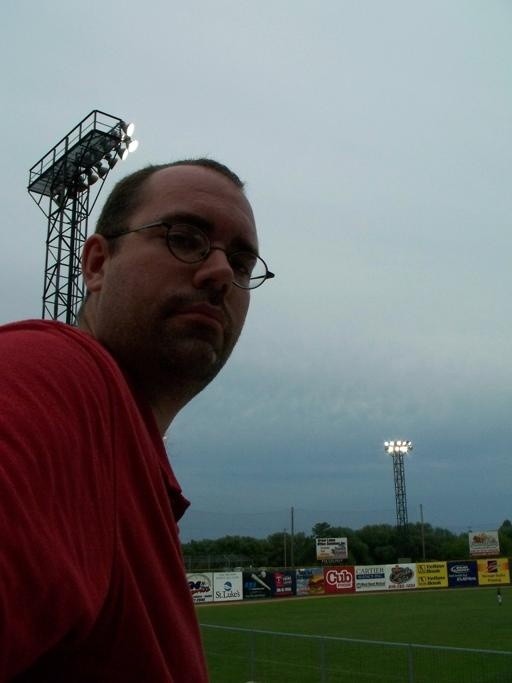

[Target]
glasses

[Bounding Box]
[108,220,274,289]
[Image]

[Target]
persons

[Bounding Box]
[0,158,258,683]
[497,588,503,606]
